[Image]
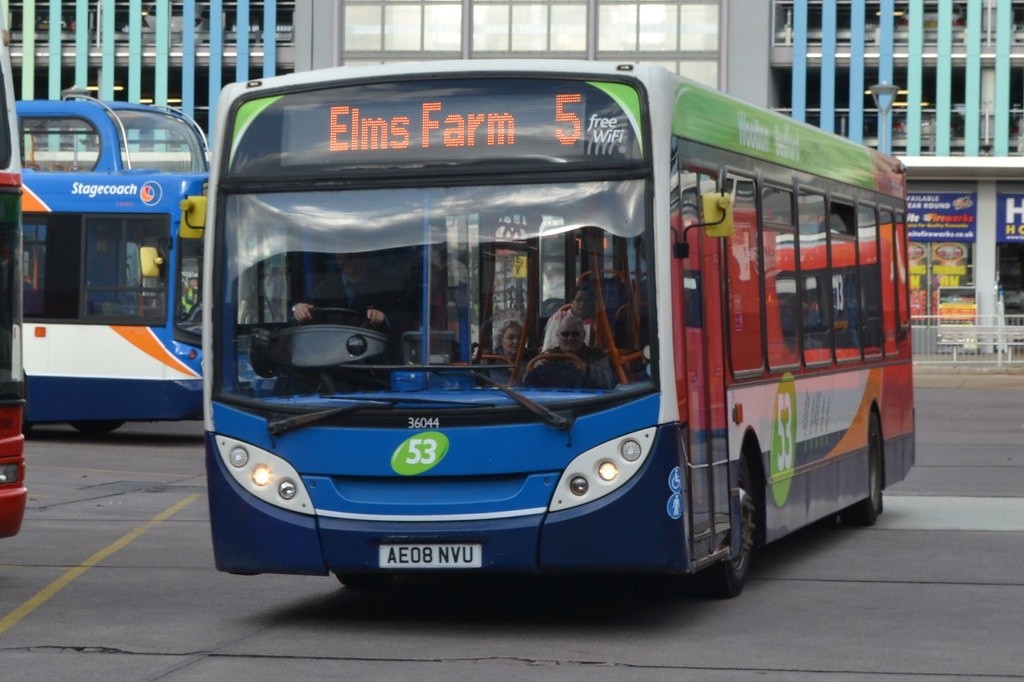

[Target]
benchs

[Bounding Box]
[936,325,1024,364]
[25,163,82,173]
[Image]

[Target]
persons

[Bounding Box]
[291,230,393,335]
[181,273,199,314]
[544,289,595,354]
[520,316,615,390]
[488,320,533,388]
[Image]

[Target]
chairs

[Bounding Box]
[472,346,637,388]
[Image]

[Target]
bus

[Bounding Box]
[671,212,914,438]
[15,95,240,435]
[0,42,28,538]
[176,59,917,606]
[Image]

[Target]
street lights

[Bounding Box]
[867,81,901,156]
[60,85,92,102]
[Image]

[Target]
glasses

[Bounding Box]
[556,330,584,338]
